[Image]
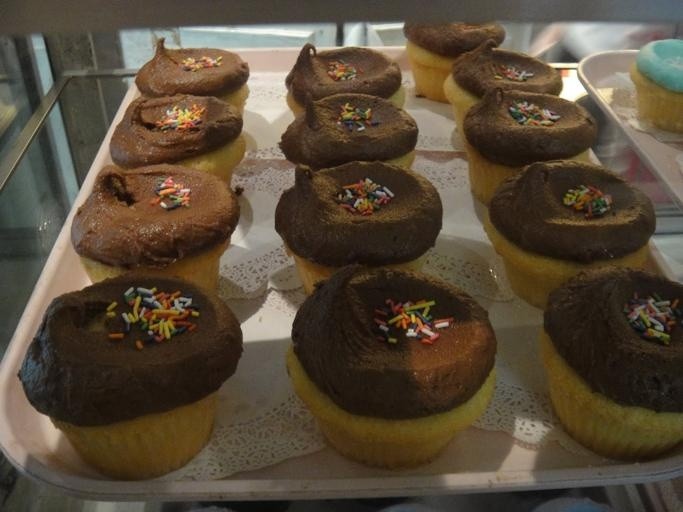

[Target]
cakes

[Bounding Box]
[488,160,657,308]
[70,165,240,300]
[404,19,505,100]
[465,91,596,202]
[18,276,242,481]
[108,95,246,183]
[445,49,562,141]
[278,94,418,180]
[630,39,682,131]
[275,162,443,297]
[540,268,682,460]
[135,39,249,111]
[286,44,405,116]
[289,268,501,469]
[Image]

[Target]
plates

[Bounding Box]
[578,48,682,210]
